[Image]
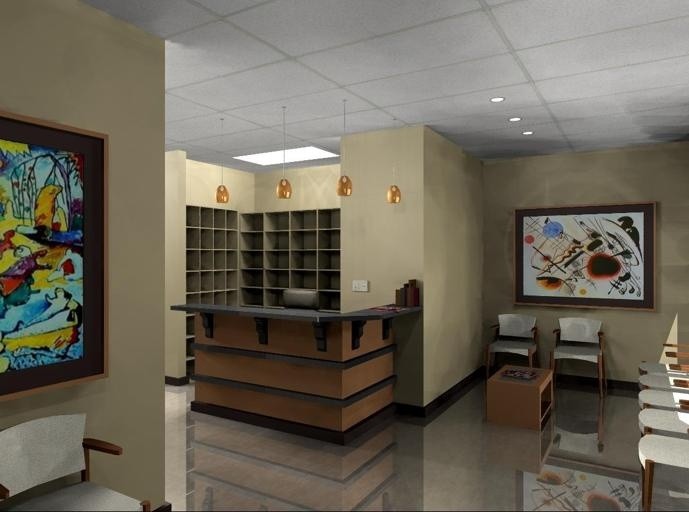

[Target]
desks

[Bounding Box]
[486,424,557,475]
[485,364,556,432]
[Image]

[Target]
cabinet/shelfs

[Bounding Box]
[186,203,239,380]
[237,207,339,313]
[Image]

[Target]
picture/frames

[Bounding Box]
[513,200,657,311]
[0,108,109,403]
[517,453,643,512]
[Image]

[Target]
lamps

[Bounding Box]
[276,105,293,200]
[387,104,403,203]
[216,117,231,204]
[335,100,352,195]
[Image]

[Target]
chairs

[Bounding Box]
[638,342,688,511]
[280,287,319,312]
[552,389,607,454]
[0,413,152,512]
[484,313,540,390]
[549,317,607,398]
